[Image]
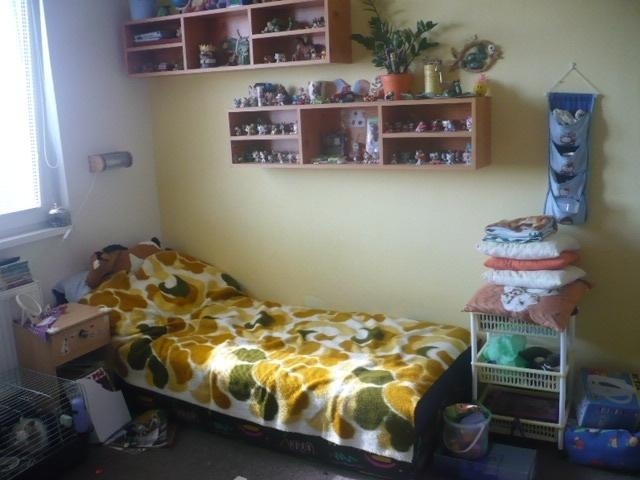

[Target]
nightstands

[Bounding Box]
[13,302,110,392]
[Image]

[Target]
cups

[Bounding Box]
[423,58,443,96]
[255,83,265,107]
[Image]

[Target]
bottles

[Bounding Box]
[70,398,92,434]
[49,207,70,228]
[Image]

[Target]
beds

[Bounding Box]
[52,238,478,480]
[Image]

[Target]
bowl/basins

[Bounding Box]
[309,80,334,101]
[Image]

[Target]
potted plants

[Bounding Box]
[349,1,442,99]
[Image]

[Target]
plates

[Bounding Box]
[518,346,556,368]
[1,455,19,473]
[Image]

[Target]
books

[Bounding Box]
[0,256,35,292]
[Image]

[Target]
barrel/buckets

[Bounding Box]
[443,404,492,458]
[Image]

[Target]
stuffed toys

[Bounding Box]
[83,234,172,290]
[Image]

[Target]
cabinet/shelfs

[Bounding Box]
[468,303,578,451]
[229,98,492,171]
[122,1,352,77]
